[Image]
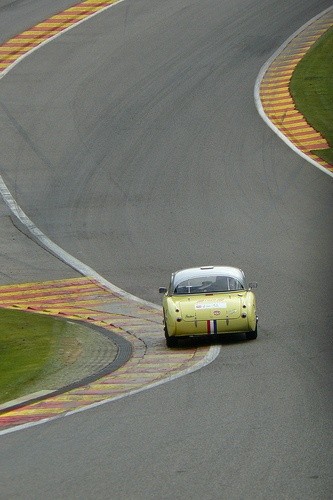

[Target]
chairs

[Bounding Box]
[202,281,214,291]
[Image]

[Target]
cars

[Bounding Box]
[160,266,259,343]
